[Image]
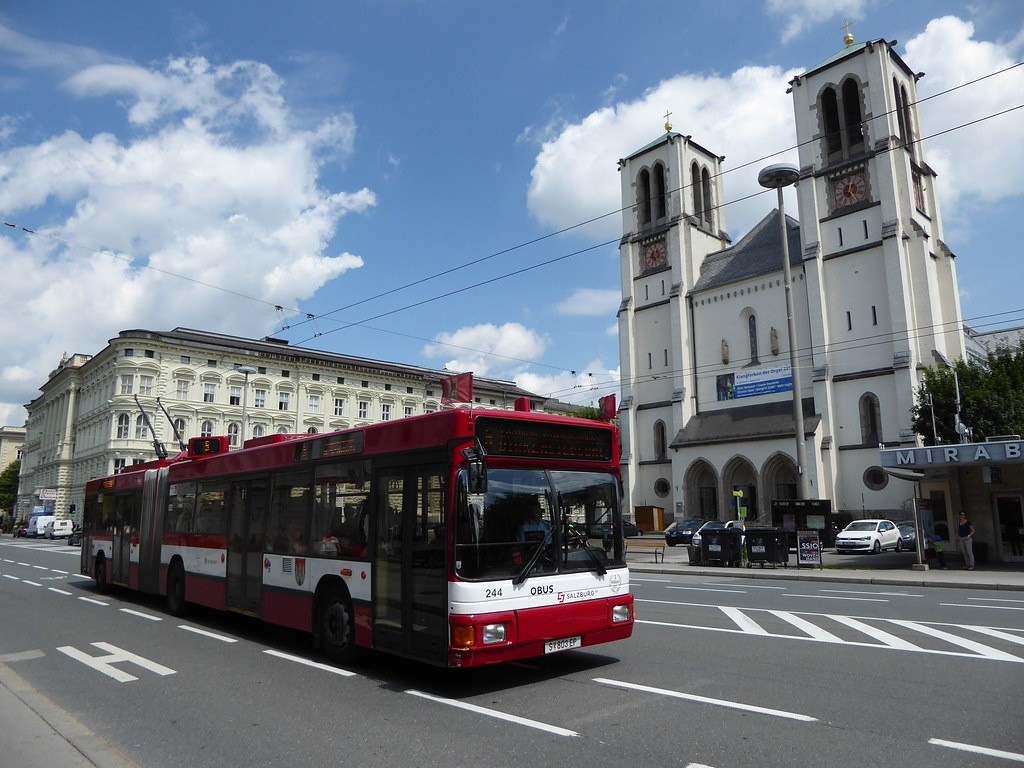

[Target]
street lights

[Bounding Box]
[758,163,815,498]
[236,365,256,452]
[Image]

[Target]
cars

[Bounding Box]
[663,519,712,547]
[601,519,644,537]
[898,523,916,552]
[834,518,903,554]
[692,521,726,549]
[12,524,28,539]
[68,526,83,547]
[723,520,790,560]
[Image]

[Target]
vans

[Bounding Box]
[44,520,74,539]
[25,516,55,539]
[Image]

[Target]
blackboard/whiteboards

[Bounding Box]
[797,531,823,568]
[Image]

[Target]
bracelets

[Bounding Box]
[968,535,970,537]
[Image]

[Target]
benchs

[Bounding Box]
[625,539,667,563]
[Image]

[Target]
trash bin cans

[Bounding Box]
[699,527,743,566]
[746,527,789,570]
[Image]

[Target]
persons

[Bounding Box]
[928,533,946,567]
[562,515,573,533]
[515,503,572,544]
[955,511,975,570]
[344,500,367,531]
[719,376,729,401]
[1002,504,1023,556]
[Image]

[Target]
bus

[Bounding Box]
[80,366,638,669]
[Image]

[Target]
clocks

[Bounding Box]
[642,238,668,272]
[824,160,873,216]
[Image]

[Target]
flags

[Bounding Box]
[598,394,616,420]
[440,373,472,404]
